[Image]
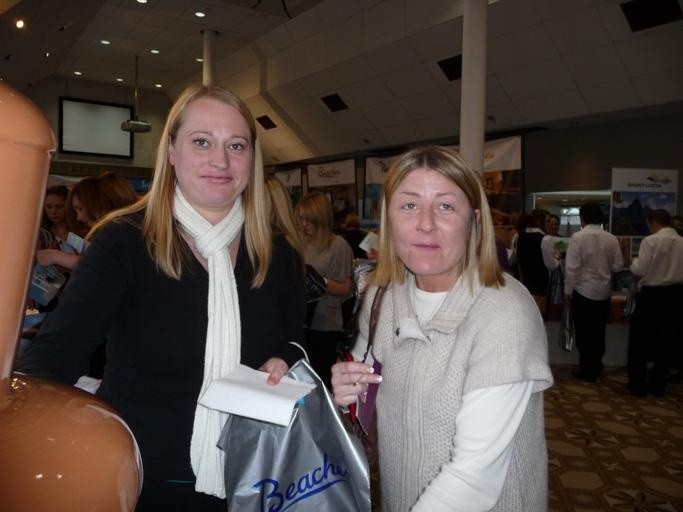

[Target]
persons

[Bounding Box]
[510,210,561,316]
[263,174,368,391]
[564,202,623,384]
[16,81,314,511]
[625,209,681,399]
[329,142,555,512]
[36,169,146,271]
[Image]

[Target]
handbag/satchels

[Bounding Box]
[559,327,573,352]
